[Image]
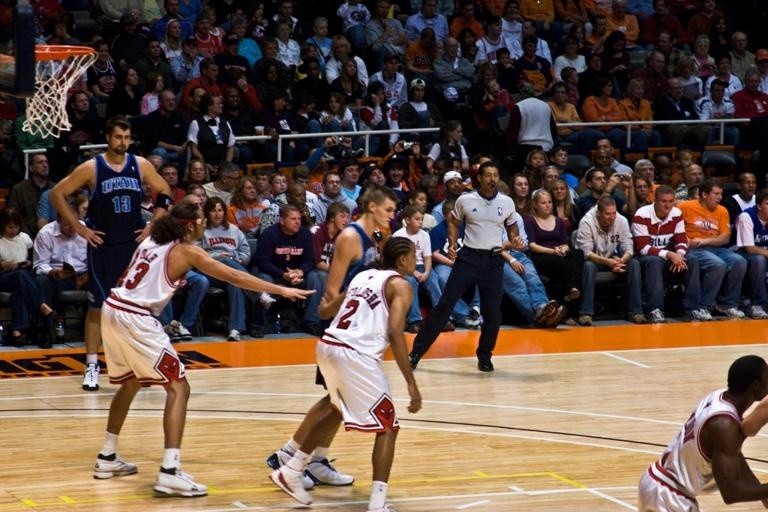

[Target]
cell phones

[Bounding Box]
[15,261,28,270]
[63,262,74,272]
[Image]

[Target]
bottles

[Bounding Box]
[272,311,282,334]
[54,319,65,344]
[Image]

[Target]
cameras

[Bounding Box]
[403,142,415,150]
[335,137,345,143]
[614,174,625,182]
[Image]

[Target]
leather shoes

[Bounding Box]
[11,310,56,348]
[250,327,264,338]
[408,353,421,369]
[259,291,276,304]
[478,359,494,372]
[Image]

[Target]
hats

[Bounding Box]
[443,170,462,186]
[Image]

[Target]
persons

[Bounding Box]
[269,236,422,511]
[1,1,767,350]
[94,200,318,498]
[49,119,172,389]
[638,356,768,512]
[740,395,768,436]
[267,191,397,490]
[411,161,524,370]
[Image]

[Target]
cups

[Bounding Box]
[254,124,264,139]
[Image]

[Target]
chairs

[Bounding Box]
[0,291,13,345]
[177,287,225,337]
[590,145,735,169]
[244,156,379,178]
[53,290,89,344]
[570,229,623,319]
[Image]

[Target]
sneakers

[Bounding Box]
[406,322,421,334]
[153,467,208,496]
[266,448,354,505]
[630,304,764,329]
[443,306,483,331]
[163,320,192,343]
[82,363,100,391]
[226,330,241,341]
[534,288,592,329]
[93,452,137,479]
